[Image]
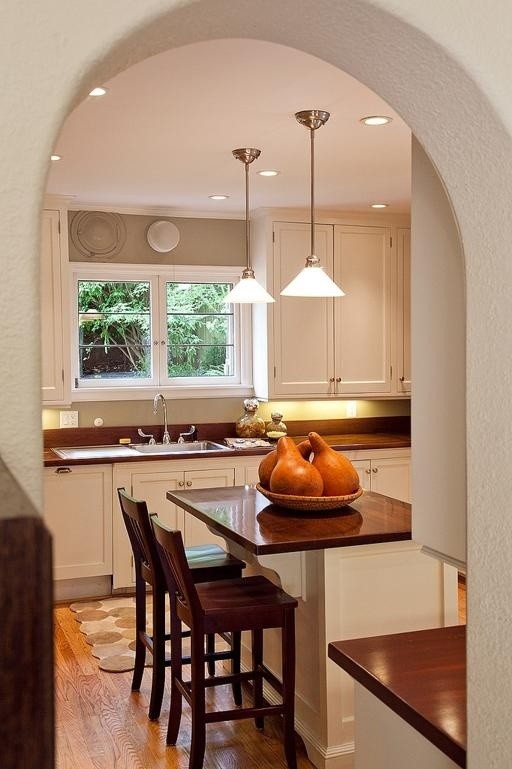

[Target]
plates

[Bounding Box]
[256,482,363,512]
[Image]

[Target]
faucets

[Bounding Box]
[153,392,172,444]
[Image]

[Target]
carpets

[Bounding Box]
[68,602,237,673]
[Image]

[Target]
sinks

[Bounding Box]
[131,441,226,454]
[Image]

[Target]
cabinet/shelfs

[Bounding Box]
[390,209,411,401]
[44,464,113,602]
[246,205,391,401]
[111,459,235,595]
[240,446,412,504]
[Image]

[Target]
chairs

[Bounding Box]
[115,487,247,720]
[150,516,299,769]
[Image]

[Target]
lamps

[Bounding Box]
[282,113,346,296]
[220,146,278,306]
